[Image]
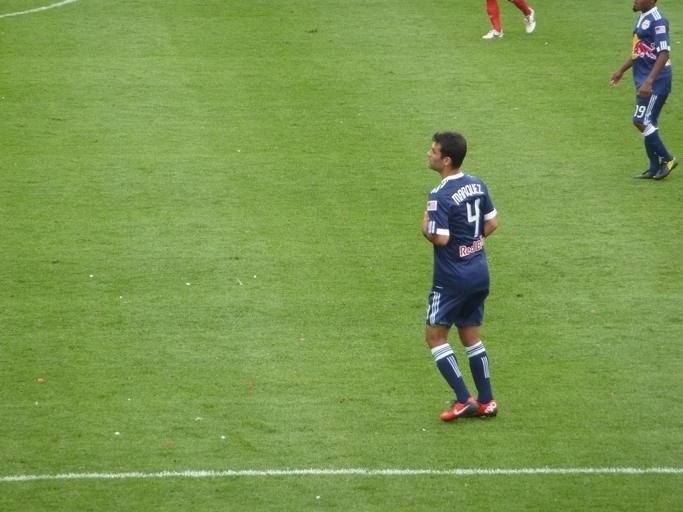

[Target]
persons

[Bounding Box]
[481,0,536,40]
[420,131,498,420]
[609,0,678,181]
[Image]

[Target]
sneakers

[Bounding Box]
[481,28,504,40]
[439,397,498,421]
[633,156,678,180]
[524,6,536,33]
